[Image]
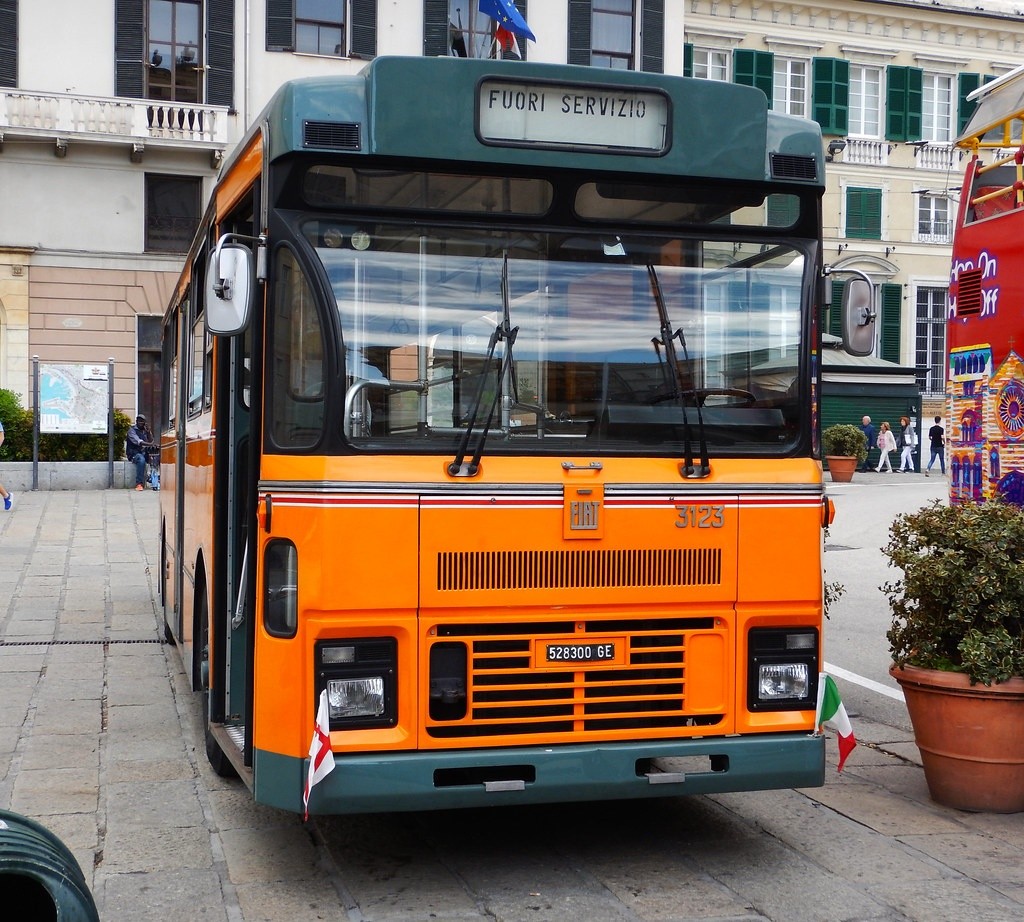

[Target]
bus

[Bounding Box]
[951,64,1023,505]
[159,57,876,820]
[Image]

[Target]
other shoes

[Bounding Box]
[925,469,930,477]
[941,473,946,475]
[859,469,866,473]
[869,465,875,472]
[896,468,905,473]
[905,470,915,473]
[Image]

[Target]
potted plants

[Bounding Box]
[821,423,869,483]
[879,489,1024,813]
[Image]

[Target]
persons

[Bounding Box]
[0,422,13,511]
[926,416,945,477]
[860,415,914,473]
[126,414,154,491]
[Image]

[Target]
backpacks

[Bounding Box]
[146,444,160,466]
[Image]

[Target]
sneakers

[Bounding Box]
[874,467,880,473]
[135,484,144,491]
[3,492,14,511]
[885,469,893,474]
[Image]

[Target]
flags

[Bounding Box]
[817,674,857,771]
[302,692,335,822]
[479,0,536,60]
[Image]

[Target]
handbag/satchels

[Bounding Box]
[905,434,918,445]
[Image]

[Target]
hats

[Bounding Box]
[137,414,146,420]
[881,421,891,429]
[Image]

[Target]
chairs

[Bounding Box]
[974,185,1017,221]
[598,331,684,447]
